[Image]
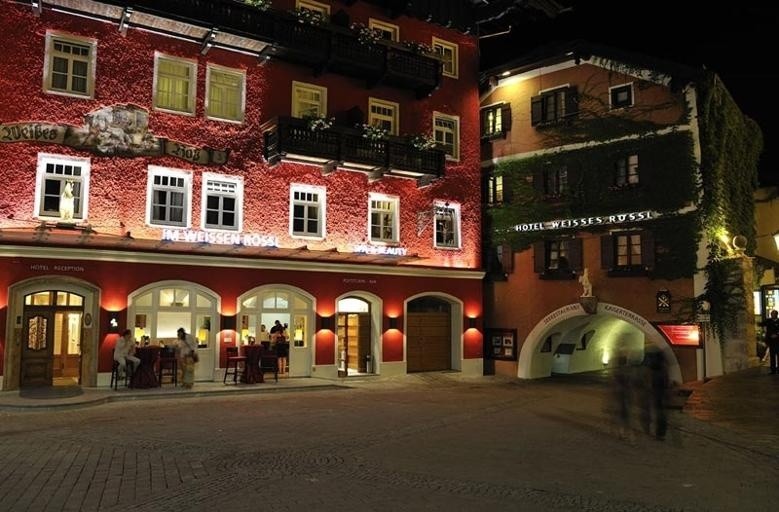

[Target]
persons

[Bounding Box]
[764,309,779,375]
[175,327,200,390]
[606,339,676,445]
[113,328,141,378]
[259,318,289,377]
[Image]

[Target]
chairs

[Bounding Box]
[112,343,279,387]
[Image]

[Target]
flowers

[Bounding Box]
[310,114,436,152]
[244,0,434,55]
